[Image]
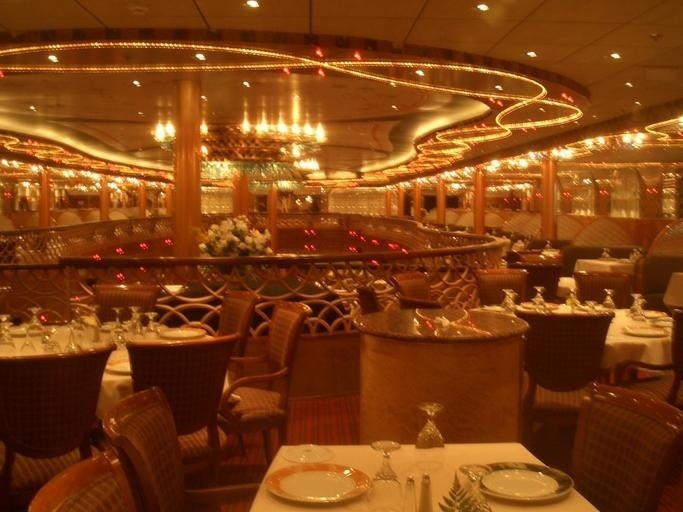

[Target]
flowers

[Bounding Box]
[197,216,273,258]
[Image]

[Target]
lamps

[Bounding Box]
[440,167,477,194]
[399,182,410,192]
[483,156,530,172]
[528,136,610,163]
[107,175,142,197]
[415,176,437,190]
[147,180,170,201]
[149,120,327,164]
[0,158,43,187]
[52,167,103,196]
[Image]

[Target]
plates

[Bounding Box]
[160,327,206,339]
[623,326,668,337]
[479,462,574,503]
[265,463,372,505]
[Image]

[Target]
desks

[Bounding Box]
[353,309,529,443]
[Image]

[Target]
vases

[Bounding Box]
[220,260,247,277]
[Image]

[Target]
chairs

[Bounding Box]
[357,286,381,314]
[508,249,525,265]
[665,308,683,404]
[391,272,432,300]
[1,343,116,504]
[216,301,313,466]
[575,272,631,308]
[639,255,683,309]
[569,382,682,511]
[28,450,141,511]
[92,283,161,327]
[573,270,633,311]
[2,261,74,325]
[512,308,615,412]
[396,295,441,309]
[218,290,260,357]
[475,268,528,306]
[103,386,260,512]
[125,332,242,467]
[507,262,562,304]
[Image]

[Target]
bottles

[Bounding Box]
[419,472,432,512]
[403,474,416,512]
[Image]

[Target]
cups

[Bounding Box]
[0,322,15,353]
[66,324,80,352]
[415,402,445,470]
[128,312,143,341]
[45,327,60,353]
[502,289,514,307]
[631,299,646,324]
[367,441,402,507]
[504,293,518,312]
[533,286,545,303]
[630,294,642,316]
[28,307,43,336]
[19,325,36,355]
[110,307,126,335]
[144,312,160,341]
[0,314,11,337]
[531,297,545,313]
[127,305,143,332]
[602,288,615,311]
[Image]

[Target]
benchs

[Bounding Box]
[561,245,646,273]
